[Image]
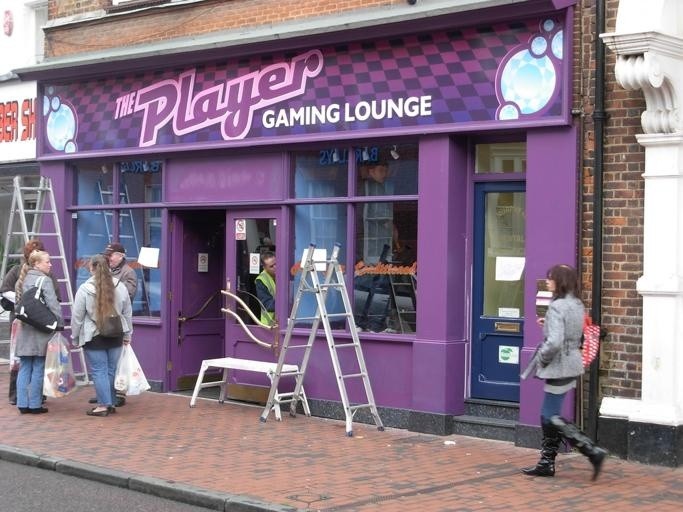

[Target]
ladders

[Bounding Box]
[260,243,386,437]
[0,176,90,385]
[361,246,417,334]
[97,182,152,316]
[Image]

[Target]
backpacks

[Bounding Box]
[81,287,124,338]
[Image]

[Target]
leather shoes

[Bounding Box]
[115,397,126,407]
[87,408,109,416]
[109,407,116,414]
[89,398,98,404]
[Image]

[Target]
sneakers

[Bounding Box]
[19,407,33,414]
[31,407,49,414]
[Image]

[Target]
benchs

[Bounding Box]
[190,356,311,421]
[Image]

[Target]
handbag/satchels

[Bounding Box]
[579,312,600,368]
[15,275,59,334]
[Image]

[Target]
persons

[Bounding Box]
[89,242,138,407]
[14,252,64,412]
[0,240,62,404]
[352,160,398,260]
[519,265,609,481]
[70,254,134,418]
[254,254,281,329]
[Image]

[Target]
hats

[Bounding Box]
[97,244,125,256]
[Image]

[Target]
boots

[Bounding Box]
[521,417,562,478]
[548,413,609,482]
